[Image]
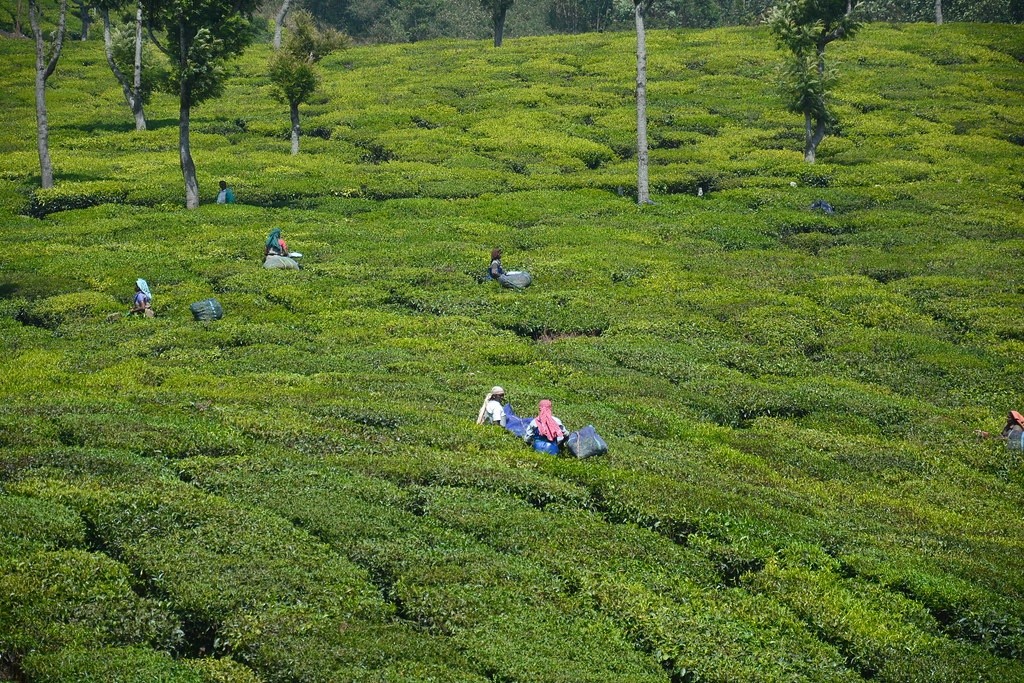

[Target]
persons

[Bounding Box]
[524,400,569,456]
[488,248,506,280]
[130,278,152,313]
[214,181,233,203]
[476,386,506,427]
[264,229,289,261]
[974,411,1024,449]
